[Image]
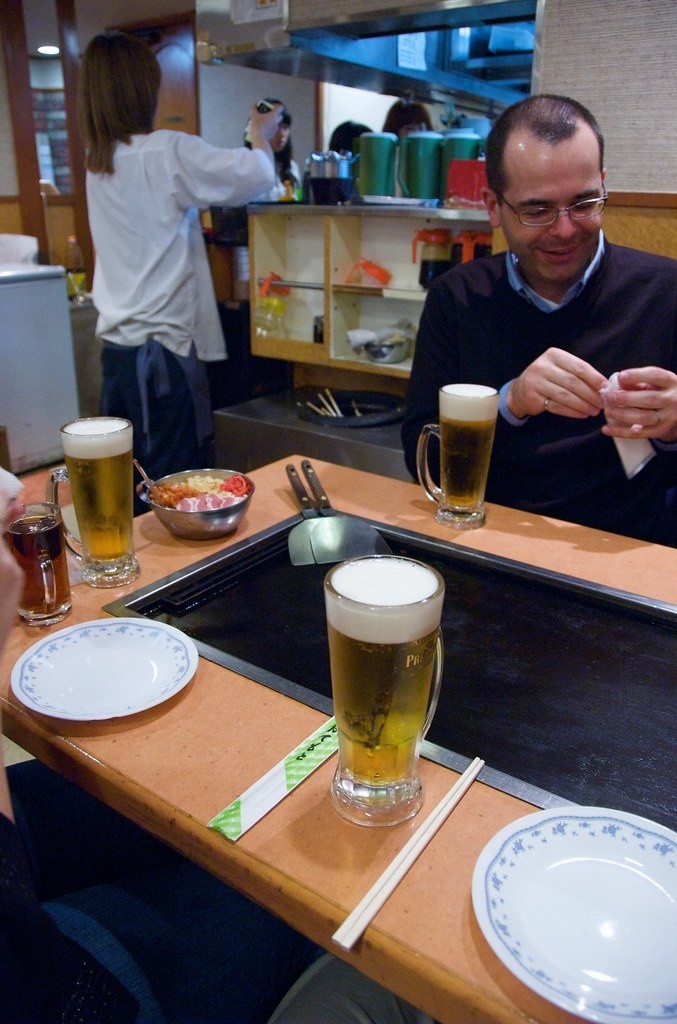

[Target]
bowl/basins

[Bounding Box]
[364,341,410,363]
[309,176,355,204]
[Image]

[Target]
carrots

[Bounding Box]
[221,475,252,496]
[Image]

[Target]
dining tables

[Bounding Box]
[2,457,677,1024]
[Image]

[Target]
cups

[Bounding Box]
[324,554,444,827]
[3,501,72,627]
[45,417,141,588]
[416,384,500,530]
[136,469,256,541]
[359,131,482,200]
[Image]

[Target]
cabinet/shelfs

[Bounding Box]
[242,208,505,400]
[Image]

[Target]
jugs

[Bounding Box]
[412,228,492,291]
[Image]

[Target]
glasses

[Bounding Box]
[493,182,609,227]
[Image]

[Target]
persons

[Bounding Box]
[0,463,329,1024]
[237,98,434,204]
[400,94,677,551]
[209,96,303,412]
[77,32,285,519]
[265,952,436,1024]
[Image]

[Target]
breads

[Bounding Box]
[38,179,59,195]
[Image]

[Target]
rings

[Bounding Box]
[654,409,660,425]
[544,397,551,410]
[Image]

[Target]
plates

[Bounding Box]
[10,617,199,721]
[471,806,677,1024]
[360,194,440,207]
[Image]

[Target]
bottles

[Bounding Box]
[64,235,86,302]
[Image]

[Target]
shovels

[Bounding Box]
[302,458,394,564]
[286,464,324,566]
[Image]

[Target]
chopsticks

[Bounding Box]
[331,757,485,952]
